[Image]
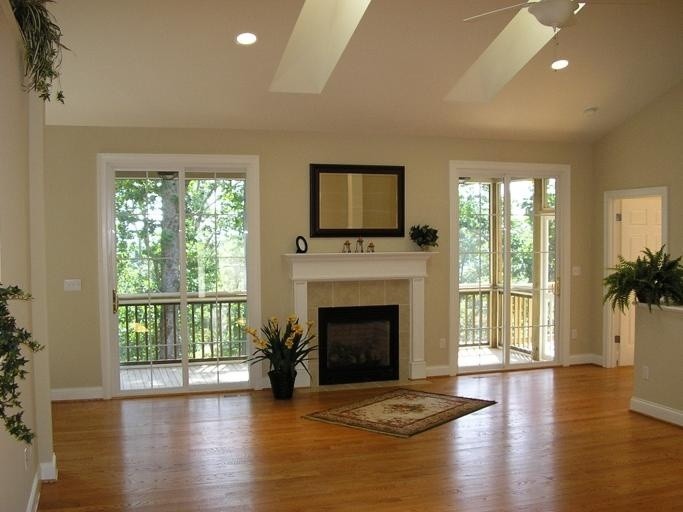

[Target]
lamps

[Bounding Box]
[526,1,582,42]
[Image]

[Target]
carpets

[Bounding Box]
[300,388,497,438]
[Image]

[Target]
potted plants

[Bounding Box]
[410,224,439,252]
[599,244,683,315]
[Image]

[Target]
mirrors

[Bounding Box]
[310,163,405,238]
[295,236,308,253]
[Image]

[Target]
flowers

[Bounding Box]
[234,313,318,370]
[267,370,297,399]
